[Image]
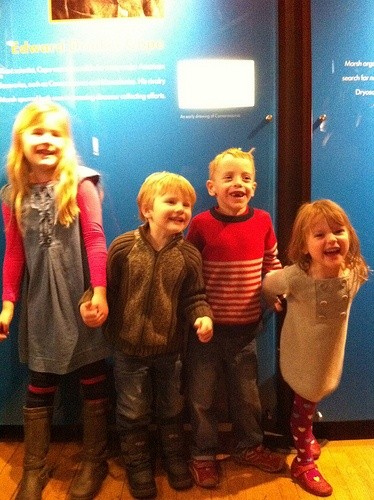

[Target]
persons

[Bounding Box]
[76,168,216,500]
[265,195,370,495]
[181,145,287,489]
[1,95,115,500]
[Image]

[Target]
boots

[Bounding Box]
[71,399,111,500]
[116,421,157,497]
[159,428,196,491]
[17,406,52,499]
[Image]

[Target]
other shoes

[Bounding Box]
[306,433,323,460]
[291,456,334,496]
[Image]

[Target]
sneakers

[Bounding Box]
[233,447,288,472]
[186,449,218,489]
